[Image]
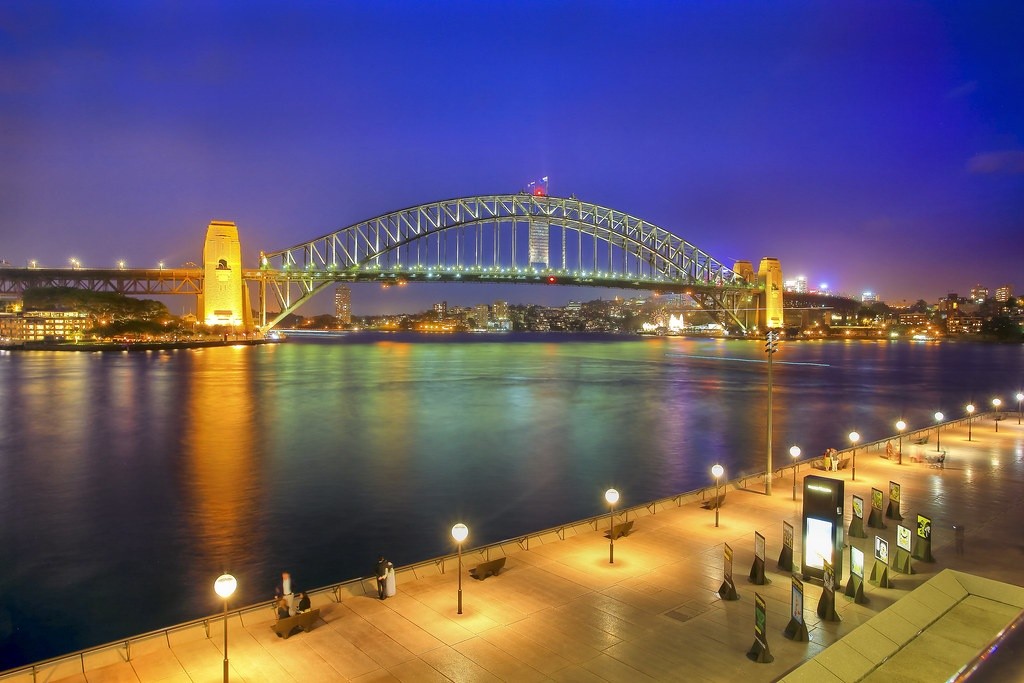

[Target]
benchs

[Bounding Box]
[702,493,726,510]
[469,556,506,582]
[837,458,850,470]
[604,520,634,541]
[277,608,320,639]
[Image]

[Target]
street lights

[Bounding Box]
[452,522,468,615]
[765,330,779,496]
[993,399,1001,432]
[712,465,724,526]
[605,489,619,563]
[1017,394,1024,424]
[967,405,975,441]
[935,412,943,452]
[790,445,800,501]
[214,573,237,683]
[897,420,906,464]
[849,432,859,480]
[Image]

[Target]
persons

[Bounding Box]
[823,447,838,472]
[375,555,396,601]
[885,440,900,460]
[296,591,311,614]
[277,572,296,620]
